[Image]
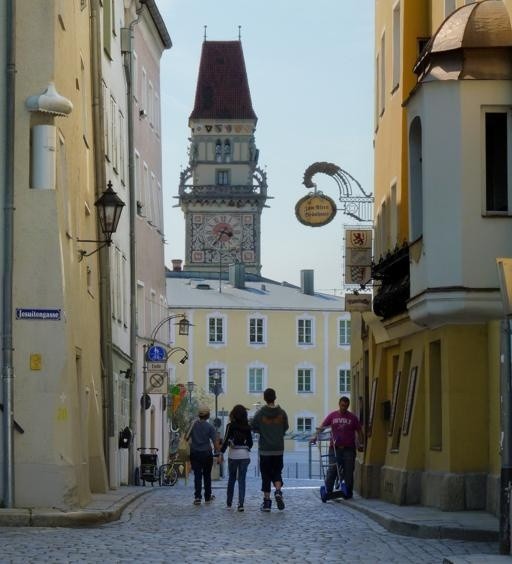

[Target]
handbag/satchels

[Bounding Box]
[177,433,190,457]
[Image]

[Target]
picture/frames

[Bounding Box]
[402,366,417,434]
[185,209,258,266]
[366,378,377,435]
[388,370,401,435]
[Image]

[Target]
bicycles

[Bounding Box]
[158,451,179,486]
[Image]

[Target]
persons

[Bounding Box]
[252,388,289,512]
[187,407,222,504]
[311,396,364,496]
[217,404,253,511]
[209,417,224,481]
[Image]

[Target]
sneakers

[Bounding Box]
[274,490,285,510]
[205,495,216,504]
[261,499,272,511]
[193,498,201,505]
[238,505,244,512]
[226,505,232,510]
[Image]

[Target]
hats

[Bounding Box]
[198,405,210,416]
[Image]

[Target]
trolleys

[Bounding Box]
[135,447,162,487]
[313,430,351,503]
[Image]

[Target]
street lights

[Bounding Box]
[185,381,196,403]
[212,370,222,431]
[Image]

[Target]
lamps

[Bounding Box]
[78,180,124,257]
